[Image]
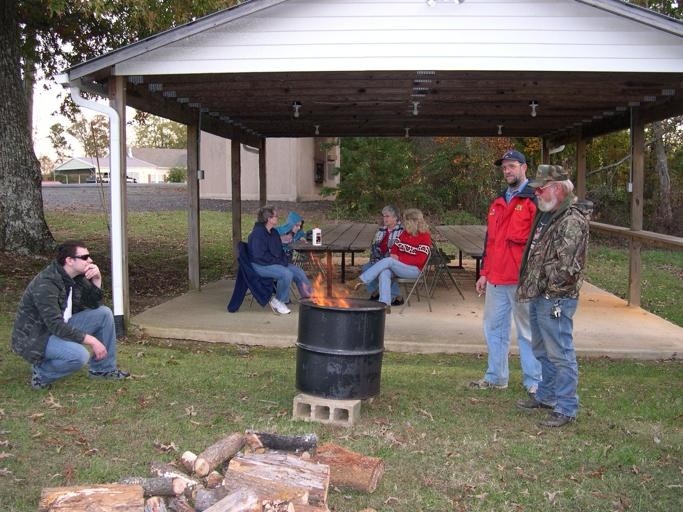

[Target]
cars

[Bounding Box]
[87,172,138,184]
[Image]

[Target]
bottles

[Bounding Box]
[312,224,322,246]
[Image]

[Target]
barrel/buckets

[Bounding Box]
[294,295,392,401]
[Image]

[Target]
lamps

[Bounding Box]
[411,101,420,116]
[292,102,302,118]
[314,124,320,135]
[496,124,504,135]
[404,127,411,138]
[529,101,539,117]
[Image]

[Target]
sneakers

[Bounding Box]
[471,378,573,428]
[87,368,130,379]
[268,296,291,314]
[345,278,404,313]
[31,364,52,390]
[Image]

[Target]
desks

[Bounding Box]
[288,224,378,298]
[434,225,488,282]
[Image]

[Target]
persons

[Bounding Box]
[515,164,590,428]
[468,151,544,398]
[345,208,432,315]
[273,212,308,294]
[10,239,132,391]
[247,205,314,314]
[361,205,404,306]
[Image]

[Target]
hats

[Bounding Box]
[528,164,568,188]
[494,150,526,166]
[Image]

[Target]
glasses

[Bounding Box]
[70,255,90,260]
[536,184,553,194]
[500,162,522,171]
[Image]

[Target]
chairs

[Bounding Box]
[395,238,466,316]
[238,241,328,314]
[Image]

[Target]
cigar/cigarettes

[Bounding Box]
[478,293,482,298]
